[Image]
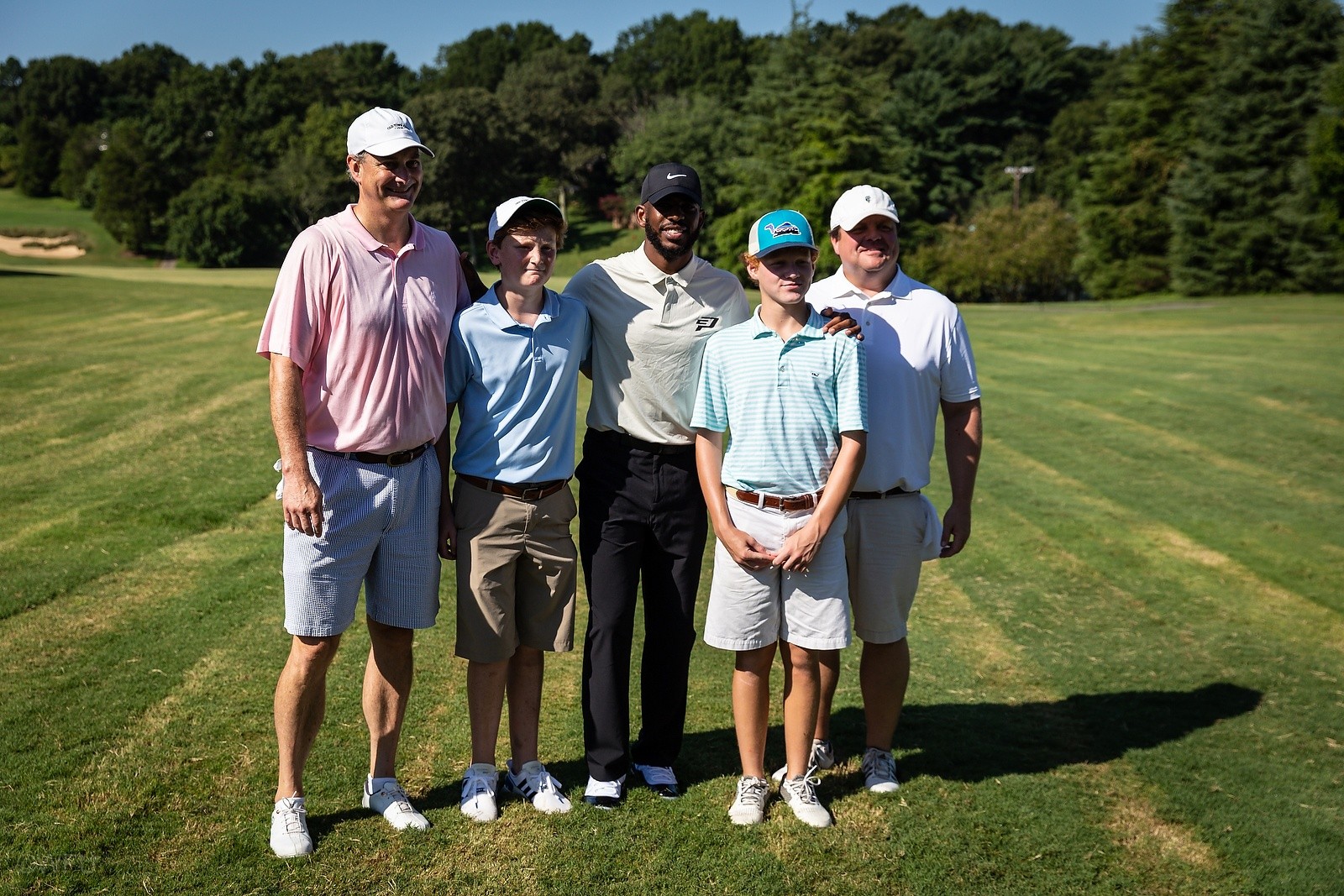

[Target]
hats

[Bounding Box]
[347,105,435,159]
[749,208,818,258]
[641,162,702,213]
[831,185,899,232]
[489,194,566,242]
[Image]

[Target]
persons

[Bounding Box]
[257,107,981,861]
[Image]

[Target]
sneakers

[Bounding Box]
[458,761,498,822]
[728,776,771,825]
[504,759,572,815]
[270,791,314,859]
[778,764,832,828]
[862,746,901,792]
[633,759,680,800]
[361,779,430,831]
[580,774,627,811]
[771,739,834,783]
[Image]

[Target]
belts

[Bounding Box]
[724,485,824,511]
[456,473,571,501]
[307,439,432,467]
[587,426,696,454]
[848,488,922,498]
